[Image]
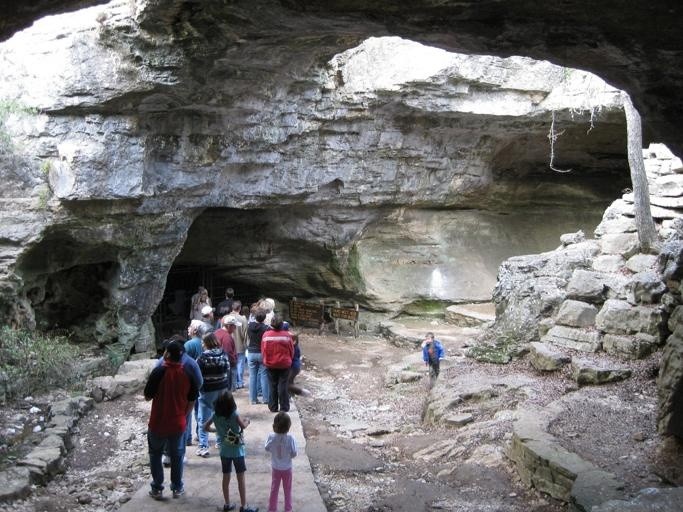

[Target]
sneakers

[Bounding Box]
[224,503,236,512]
[239,505,259,512]
[148,436,221,498]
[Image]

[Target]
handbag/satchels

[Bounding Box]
[223,429,244,446]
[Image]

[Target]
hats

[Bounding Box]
[224,315,242,327]
[163,339,184,356]
[202,305,216,317]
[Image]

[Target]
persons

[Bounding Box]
[423,331,444,386]
[140,279,301,512]
